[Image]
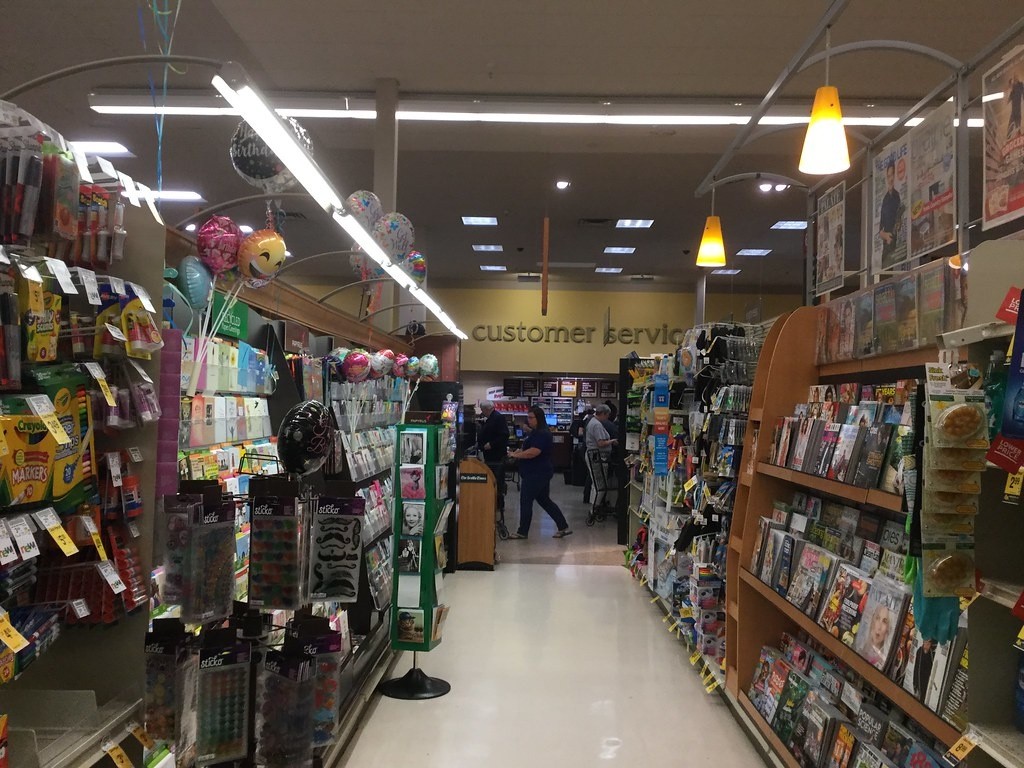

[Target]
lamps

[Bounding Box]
[695,187,726,268]
[88,86,987,128]
[318,260,421,300]
[2,52,347,214]
[407,328,470,344]
[797,27,851,174]
[359,287,444,322]
[387,309,457,333]
[175,192,393,278]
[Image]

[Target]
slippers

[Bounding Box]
[508,532,528,539]
[552,531,573,538]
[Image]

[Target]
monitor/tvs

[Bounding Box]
[545,414,558,426]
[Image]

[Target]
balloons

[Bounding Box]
[344,191,415,267]
[372,350,395,378]
[277,400,334,477]
[196,216,287,288]
[393,354,439,379]
[178,256,212,309]
[230,115,312,208]
[324,347,372,382]
[400,251,426,282]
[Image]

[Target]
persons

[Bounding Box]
[913,638,935,692]
[403,506,423,536]
[866,605,891,650]
[507,405,573,540]
[795,418,808,456]
[410,470,420,490]
[573,401,617,517]
[879,162,900,280]
[472,400,509,513]
[1006,72,1024,138]
[401,540,419,568]
[839,303,854,354]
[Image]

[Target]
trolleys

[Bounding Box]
[585,448,620,526]
[485,458,513,540]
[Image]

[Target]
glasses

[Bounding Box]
[526,416,536,420]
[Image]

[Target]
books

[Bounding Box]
[748,377,952,768]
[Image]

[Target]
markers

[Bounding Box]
[17,156,44,237]
[77,390,93,492]
[0,290,21,383]
[0,607,61,673]
[0,557,38,601]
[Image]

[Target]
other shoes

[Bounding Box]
[588,508,596,515]
[583,502,592,505]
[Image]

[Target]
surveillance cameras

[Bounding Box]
[517,248,524,251]
[683,249,690,255]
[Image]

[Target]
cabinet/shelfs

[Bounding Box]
[457,459,497,570]
[616,231,1024,768]
